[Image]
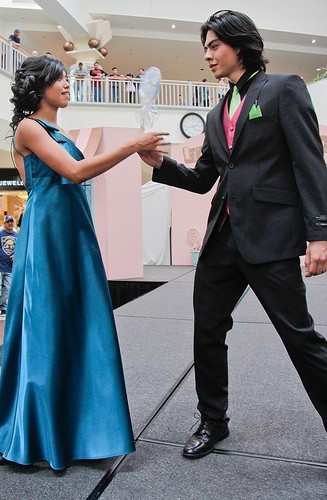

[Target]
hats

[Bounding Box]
[4,216,14,223]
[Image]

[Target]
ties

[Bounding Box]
[228,70,260,121]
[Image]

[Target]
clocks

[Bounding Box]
[180,113,206,139]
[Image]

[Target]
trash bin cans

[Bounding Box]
[192,251,200,266]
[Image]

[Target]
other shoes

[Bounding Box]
[1,310,7,317]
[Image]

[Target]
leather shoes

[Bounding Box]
[182,419,230,458]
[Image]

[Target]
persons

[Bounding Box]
[3,29,22,71]
[0,215,19,317]
[74,63,145,103]
[1,55,172,473]
[177,77,228,107]
[137,10,327,458]
